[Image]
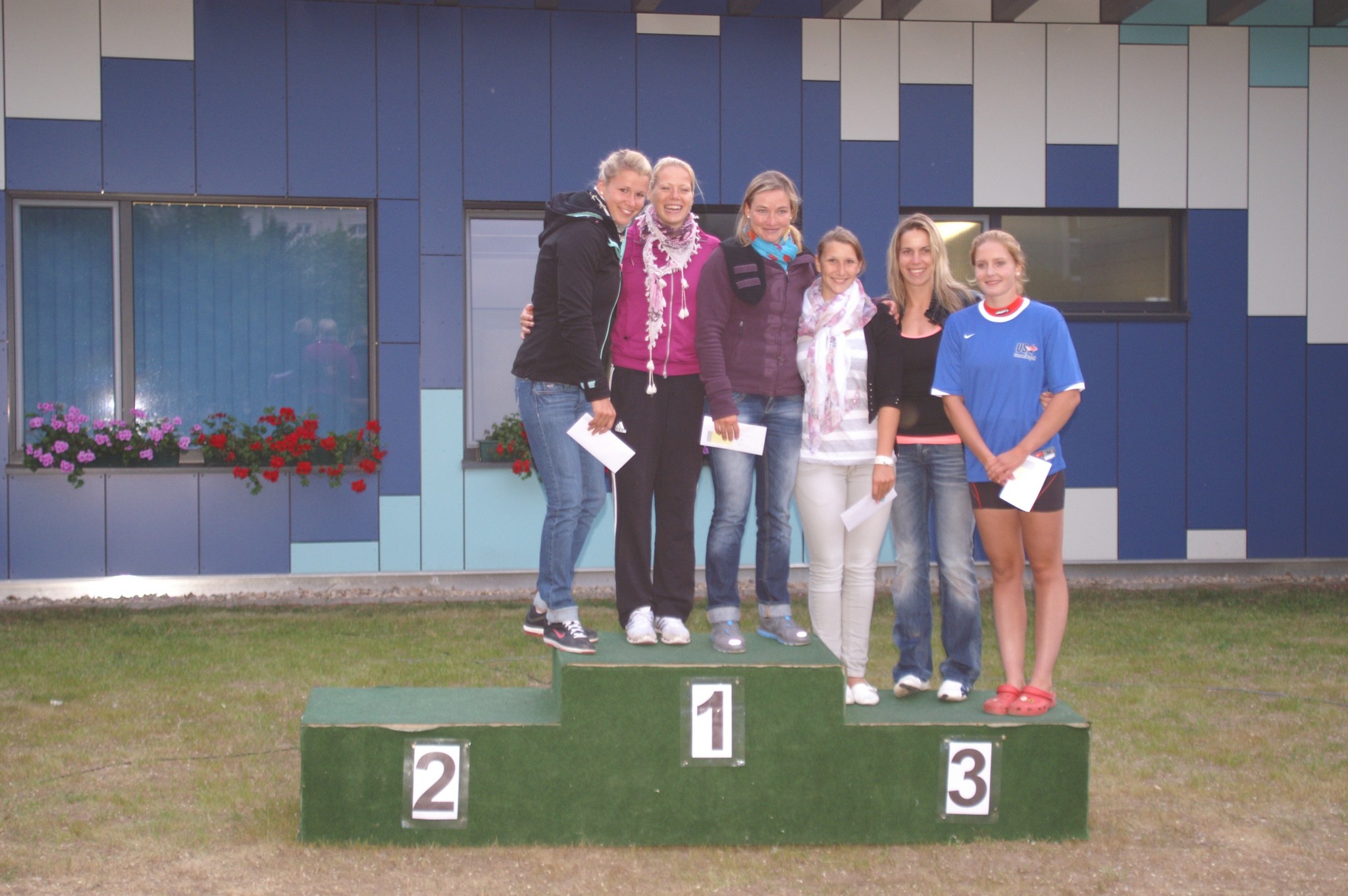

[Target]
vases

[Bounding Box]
[289,442,354,466]
[127,449,180,468]
[477,438,522,462]
[59,451,123,468]
[211,445,280,466]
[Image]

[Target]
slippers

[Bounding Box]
[983,684,1056,716]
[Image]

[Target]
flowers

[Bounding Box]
[485,416,542,479]
[16,401,388,498]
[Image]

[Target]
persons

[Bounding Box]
[510,149,652,655]
[795,227,904,706]
[887,214,1055,704]
[694,171,900,655]
[930,229,1086,717]
[519,155,721,645]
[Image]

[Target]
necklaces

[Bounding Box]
[984,294,1024,317]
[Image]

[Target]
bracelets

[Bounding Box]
[872,455,894,466]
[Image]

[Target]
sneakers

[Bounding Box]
[523,605,599,643]
[654,615,691,645]
[711,621,746,653]
[846,678,879,706]
[894,675,931,698]
[937,680,969,701]
[758,616,810,645]
[543,620,596,654]
[625,606,658,644]
